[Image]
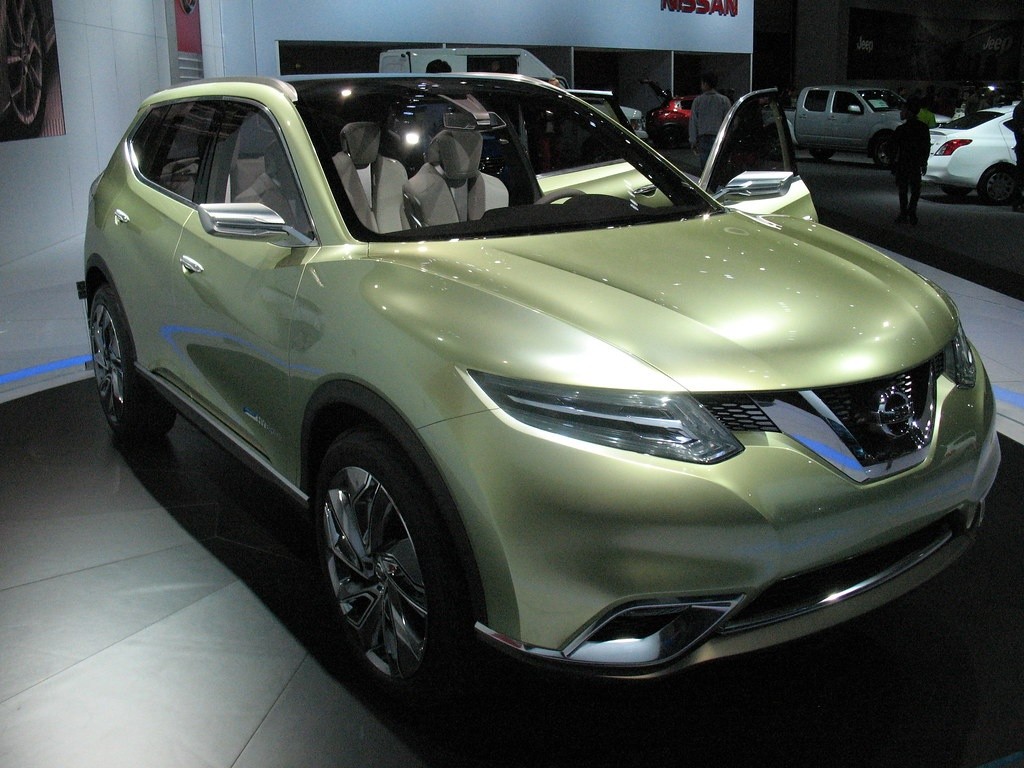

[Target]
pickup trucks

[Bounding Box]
[760,77,954,172]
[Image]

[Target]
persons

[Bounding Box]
[489,59,502,75]
[425,59,453,74]
[688,73,736,192]
[549,78,559,87]
[888,82,1024,225]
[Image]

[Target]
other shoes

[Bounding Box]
[909,206,918,225]
[895,209,909,224]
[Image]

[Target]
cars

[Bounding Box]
[643,92,702,150]
[76,70,1003,719]
[0,0,59,140]
[918,102,1024,207]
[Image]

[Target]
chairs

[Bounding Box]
[401,129,509,223]
[329,119,409,233]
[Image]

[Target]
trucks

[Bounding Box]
[377,45,650,145]
